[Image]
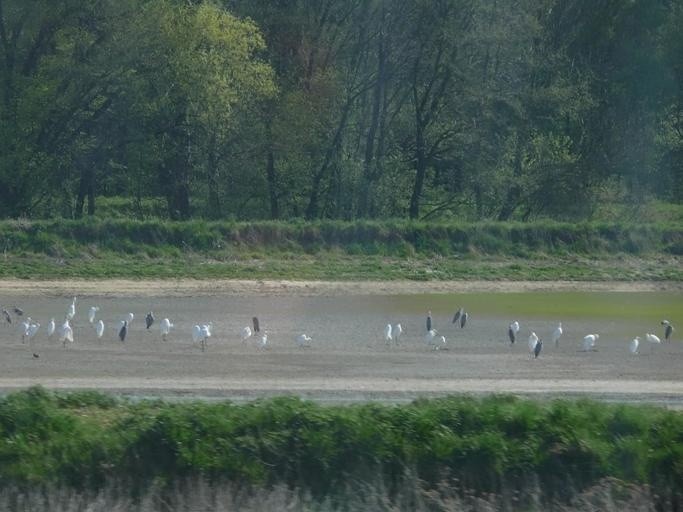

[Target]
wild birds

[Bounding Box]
[551,322,562,348]
[629,336,641,357]
[384,307,469,351]
[508,320,544,358]
[645,333,661,353]
[295,335,313,349]
[242,317,269,349]
[583,333,599,351]
[1,296,212,349]
[661,319,675,341]
